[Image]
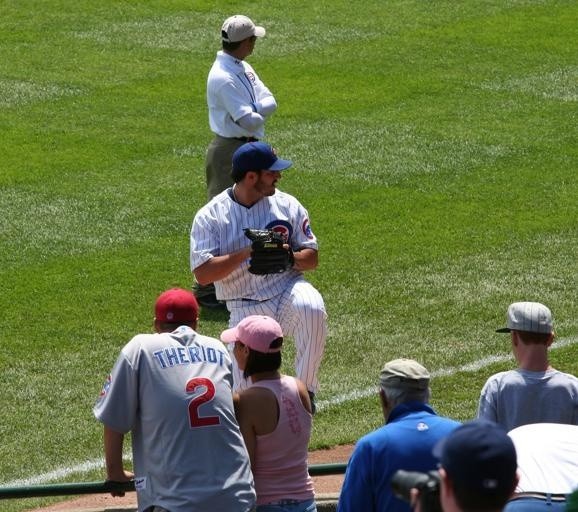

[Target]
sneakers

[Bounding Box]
[308,390,315,414]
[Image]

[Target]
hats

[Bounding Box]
[154,288,199,322]
[231,141,291,172]
[220,315,283,354]
[431,420,516,472]
[494,302,552,335]
[379,359,430,392]
[219,14,265,42]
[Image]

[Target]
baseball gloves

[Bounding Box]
[242,228,294,274]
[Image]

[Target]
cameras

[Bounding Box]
[392,469,442,512]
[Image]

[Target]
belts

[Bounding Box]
[507,493,565,503]
[264,499,297,506]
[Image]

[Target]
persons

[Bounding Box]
[336,356,465,511]
[475,300,578,511]
[219,313,319,511]
[90,288,258,511]
[187,139,328,419]
[409,419,519,511]
[191,13,278,310]
